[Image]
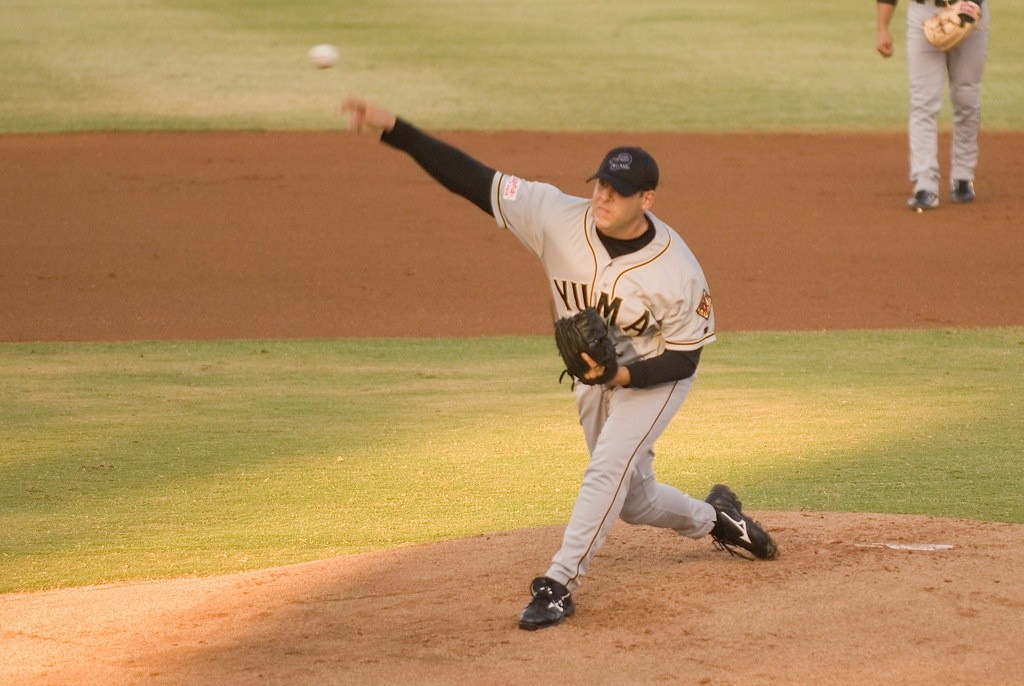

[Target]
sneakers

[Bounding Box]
[952,178,975,202]
[518,577,575,631]
[704,484,778,561]
[907,190,939,210]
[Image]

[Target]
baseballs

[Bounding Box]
[307,44,340,69]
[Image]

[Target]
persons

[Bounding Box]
[340,96,780,631]
[877,0,988,210]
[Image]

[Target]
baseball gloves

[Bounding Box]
[921,0,983,53]
[553,306,618,392]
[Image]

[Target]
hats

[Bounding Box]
[585,146,659,196]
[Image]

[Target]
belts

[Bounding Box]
[917,0,958,7]
